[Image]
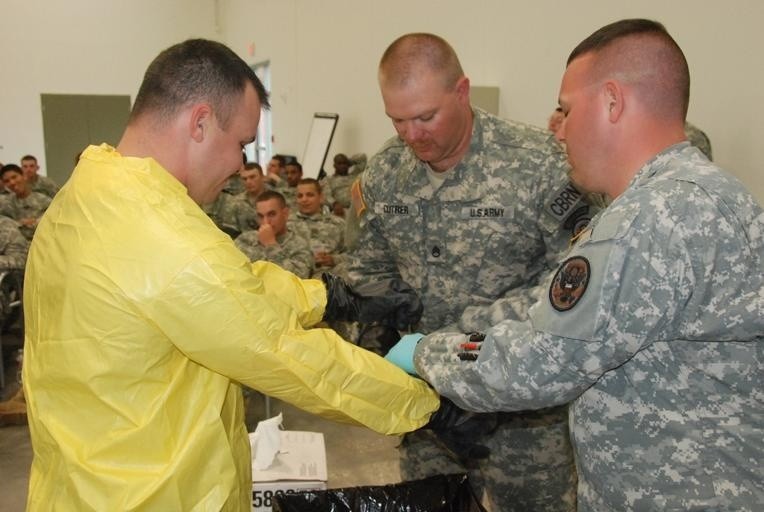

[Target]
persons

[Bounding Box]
[25,38,467,511]
[313,32,604,511]
[0,155,367,367]
[603,120,712,209]
[383,20,763,509]
[547,108,564,132]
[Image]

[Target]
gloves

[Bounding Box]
[355,324,401,359]
[321,271,424,332]
[423,394,500,442]
[383,333,426,375]
